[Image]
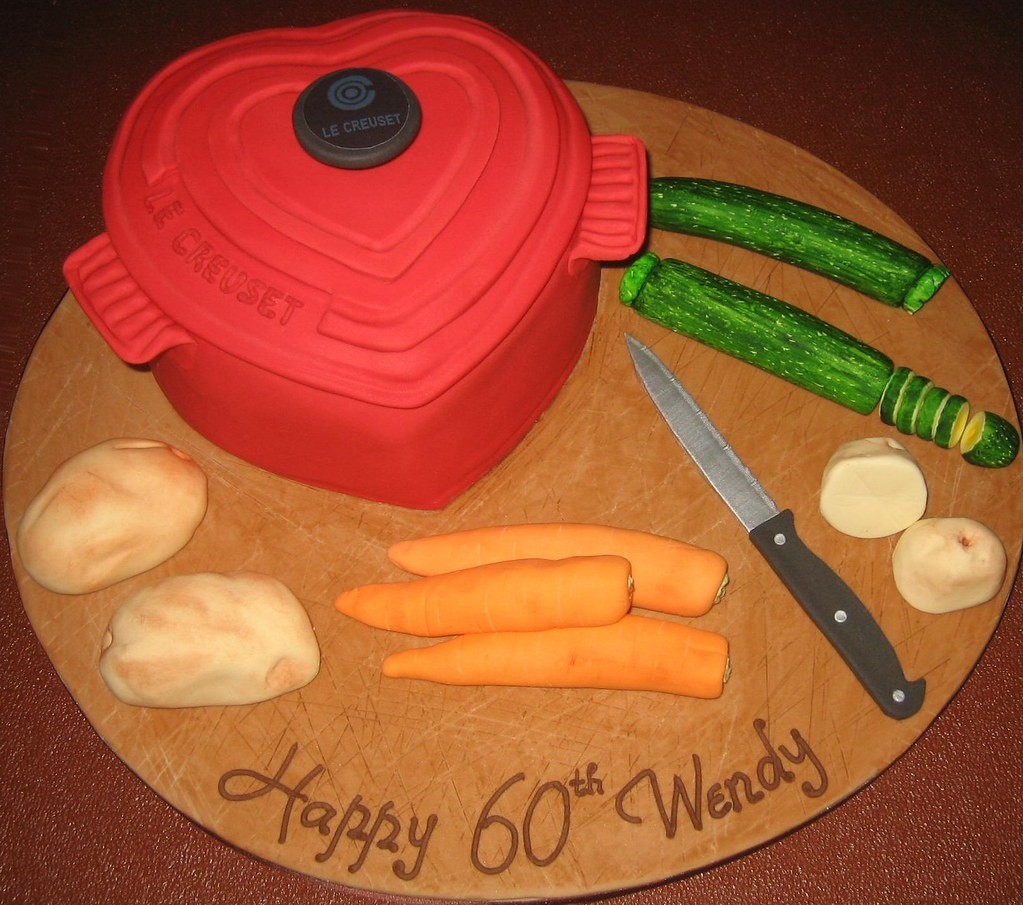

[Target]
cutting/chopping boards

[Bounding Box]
[5,89,1022,901]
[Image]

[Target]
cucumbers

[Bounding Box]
[618,177,1023,469]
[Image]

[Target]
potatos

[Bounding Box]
[18,431,326,709]
[817,435,1009,613]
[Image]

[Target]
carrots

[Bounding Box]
[335,520,733,698]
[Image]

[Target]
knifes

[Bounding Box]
[619,334,927,722]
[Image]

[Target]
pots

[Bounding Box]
[63,19,655,507]
